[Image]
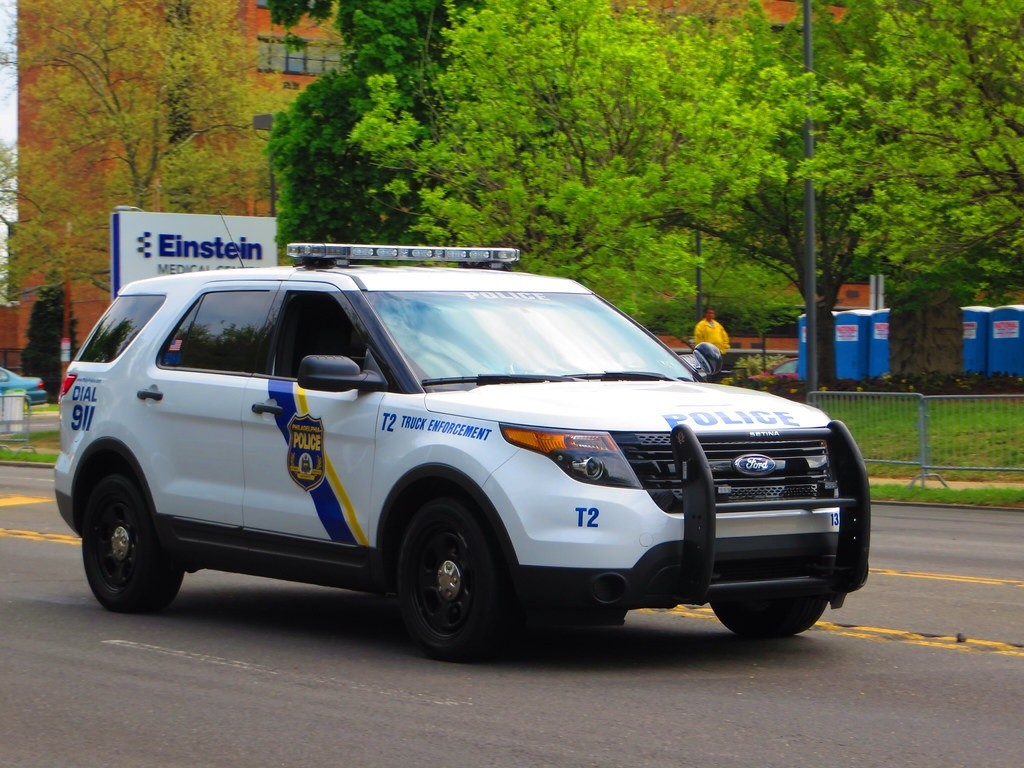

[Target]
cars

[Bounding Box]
[751,357,799,378]
[0,367,49,405]
[52,241,867,662]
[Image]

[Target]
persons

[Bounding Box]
[694,305,731,383]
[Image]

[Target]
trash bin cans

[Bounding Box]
[960,305,995,375]
[867,307,889,380]
[835,310,871,382]
[988,305,1024,378]
[799,305,836,383]
